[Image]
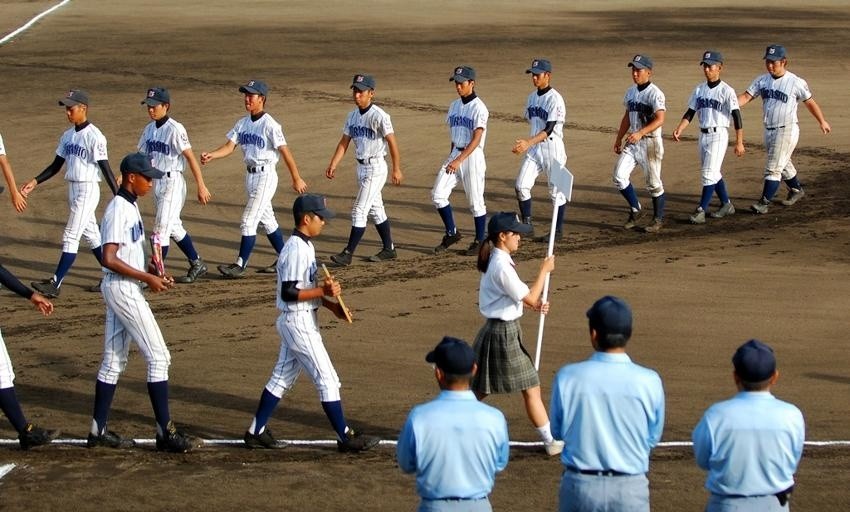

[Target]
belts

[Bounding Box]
[700,127,718,133]
[246,165,265,174]
[421,495,491,501]
[764,126,786,130]
[455,146,466,152]
[564,464,630,477]
[541,137,552,143]
[643,135,654,140]
[357,156,373,165]
[166,171,171,176]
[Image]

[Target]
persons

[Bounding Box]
[671,50,744,224]
[244,193,381,453]
[0,130,27,212]
[548,295,667,511]
[87,151,206,453]
[199,80,308,276]
[395,337,512,510]
[611,54,668,232]
[432,65,488,257]
[0,259,63,446]
[468,211,564,456]
[325,74,405,265]
[114,86,212,285]
[690,337,807,512]
[509,60,569,242]
[19,89,117,298]
[733,44,834,215]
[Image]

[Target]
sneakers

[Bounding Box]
[463,239,485,256]
[781,185,806,206]
[533,228,563,242]
[89,280,103,292]
[624,204,647,230]
[749,201,770,215]
[216,264,246,278]
[331,249,353,265]
[244,426,287,449]
[434,232,461,254]
[177,256,206,284]
[369,248,397,262]
[155,432,203,451]
[86,431,136,448]
[337,425,381,453]
[31,280,60,299]
[711,199,736,218]
[688,207,707,224]
[544,438,565,457]
[19,422,63,450]
[263,260,278,274]
[645,215,665,232]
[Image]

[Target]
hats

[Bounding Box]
[239,79,269,100]
[732,339,778,383]
[585,296,633,335]
[119,152,166,180]
[141,86,170,108]
[628,55,653,69]
[426,335,479,375]
[700,51,724,66]
[58,88,89,108]
[488,212,536,234]
[449,65,475,84]
[350,74,376,92]
[526,58,552,75]
[292,192,338,218]
[763,45,786,61]
